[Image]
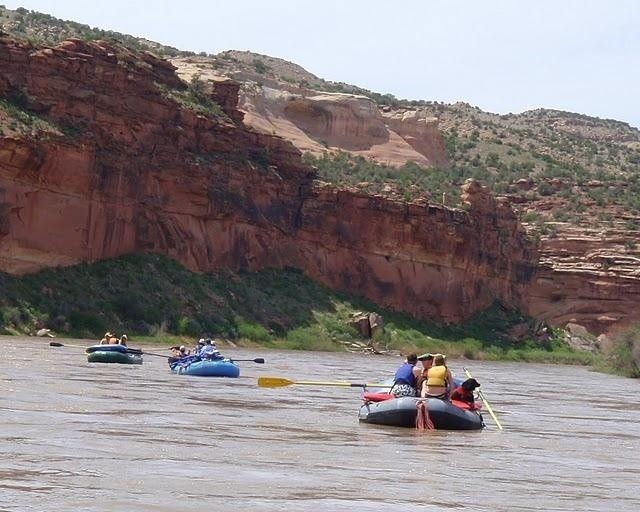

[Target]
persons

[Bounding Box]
[100,332,128,346]
[391,352,480,404]
[171,337,222,361]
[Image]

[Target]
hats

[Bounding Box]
[407,354,417,364]
[435,354,444,366]
[198,338,217,346]
[123,335,127,340]
[179,344,185,352]
[104,332,113,338]
[417,353,434,360]
[461,378,480,387]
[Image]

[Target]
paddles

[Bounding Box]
[258,377,393,388]
[141,346,180,351]
[232,358,264,363]
[49,342,86,349]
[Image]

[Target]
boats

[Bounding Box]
[358,378,486,431]
[86,344,143,364]
[169,355,239,378]
